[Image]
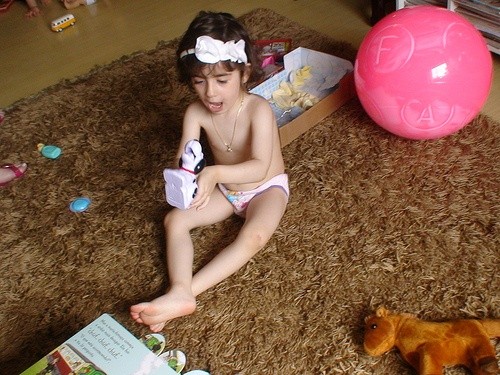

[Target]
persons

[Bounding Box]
[130,10,289,333]
[0,162,28,186]
[25,0,98,18]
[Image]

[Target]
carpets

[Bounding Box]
[0,8,500,374]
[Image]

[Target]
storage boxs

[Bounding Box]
[247,47,355,147]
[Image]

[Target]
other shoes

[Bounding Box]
[0,161,30,188]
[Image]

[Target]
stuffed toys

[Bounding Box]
[364,302,500,375]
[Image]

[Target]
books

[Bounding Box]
[21,313,181,375]
[453,0,500,49]
[404,0,447,8]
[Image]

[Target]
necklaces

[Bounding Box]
[210,91,244,152]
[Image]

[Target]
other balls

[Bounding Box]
[354,6,494,141]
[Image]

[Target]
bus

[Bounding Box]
[50,13,76,33]
